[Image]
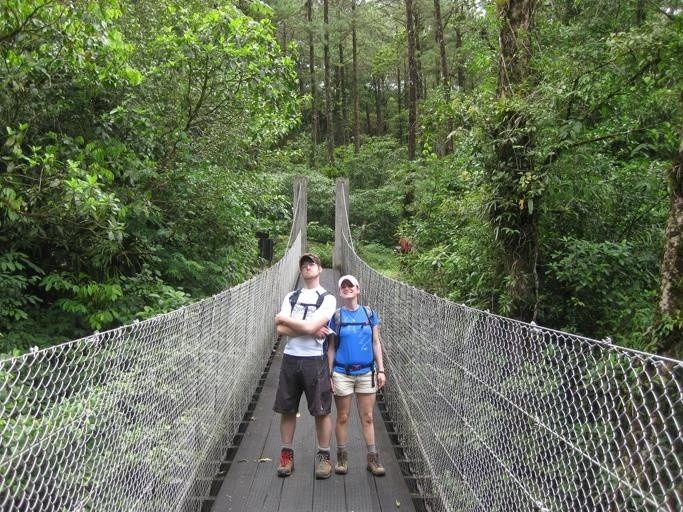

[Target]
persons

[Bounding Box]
[327,274,386,476]
[276,253,337,478]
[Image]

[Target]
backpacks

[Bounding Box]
[288,287,335,352]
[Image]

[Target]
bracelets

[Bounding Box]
[377,371,384,373]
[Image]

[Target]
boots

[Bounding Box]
[315,449,332,479]
[277,448,294,476]
[367,453,385,476]
[335,449,348,474]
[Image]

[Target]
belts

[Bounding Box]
[283,353,326,361]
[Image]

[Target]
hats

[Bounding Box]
[338,275,359,288]
[299,254,321,265]
